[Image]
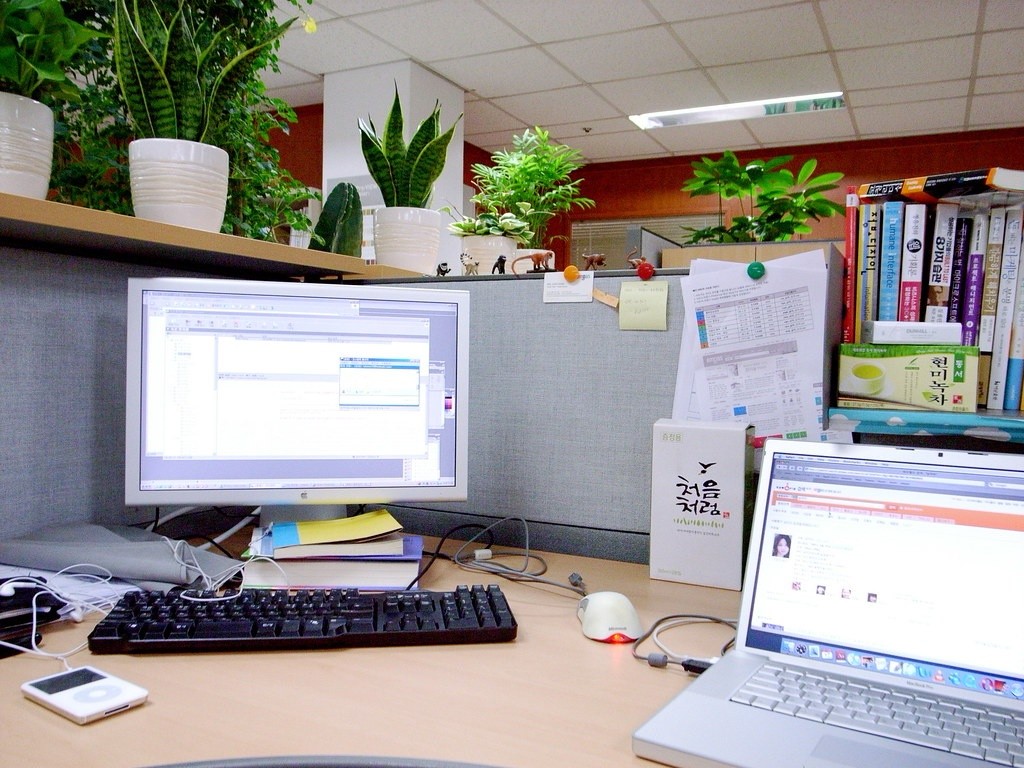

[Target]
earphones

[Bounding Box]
[69,602,84,623]
[0,582,37,596]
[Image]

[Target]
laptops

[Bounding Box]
[632,439,1024,768]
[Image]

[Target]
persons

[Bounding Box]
[773,533,791,558]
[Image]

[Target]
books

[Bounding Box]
[841,166,1024,415]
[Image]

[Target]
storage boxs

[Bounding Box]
[838,343,980,412]
[650,418,755,590]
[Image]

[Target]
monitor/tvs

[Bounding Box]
[123,278,469,557]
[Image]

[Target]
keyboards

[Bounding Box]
[86,583,520,653]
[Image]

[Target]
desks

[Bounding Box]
[0,530,742,768]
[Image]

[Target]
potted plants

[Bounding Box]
[437,125,597,274]
[358,79,464,274]
[114,0,300,234]
[0,0,118,199]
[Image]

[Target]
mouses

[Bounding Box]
[579,591,642,642]
